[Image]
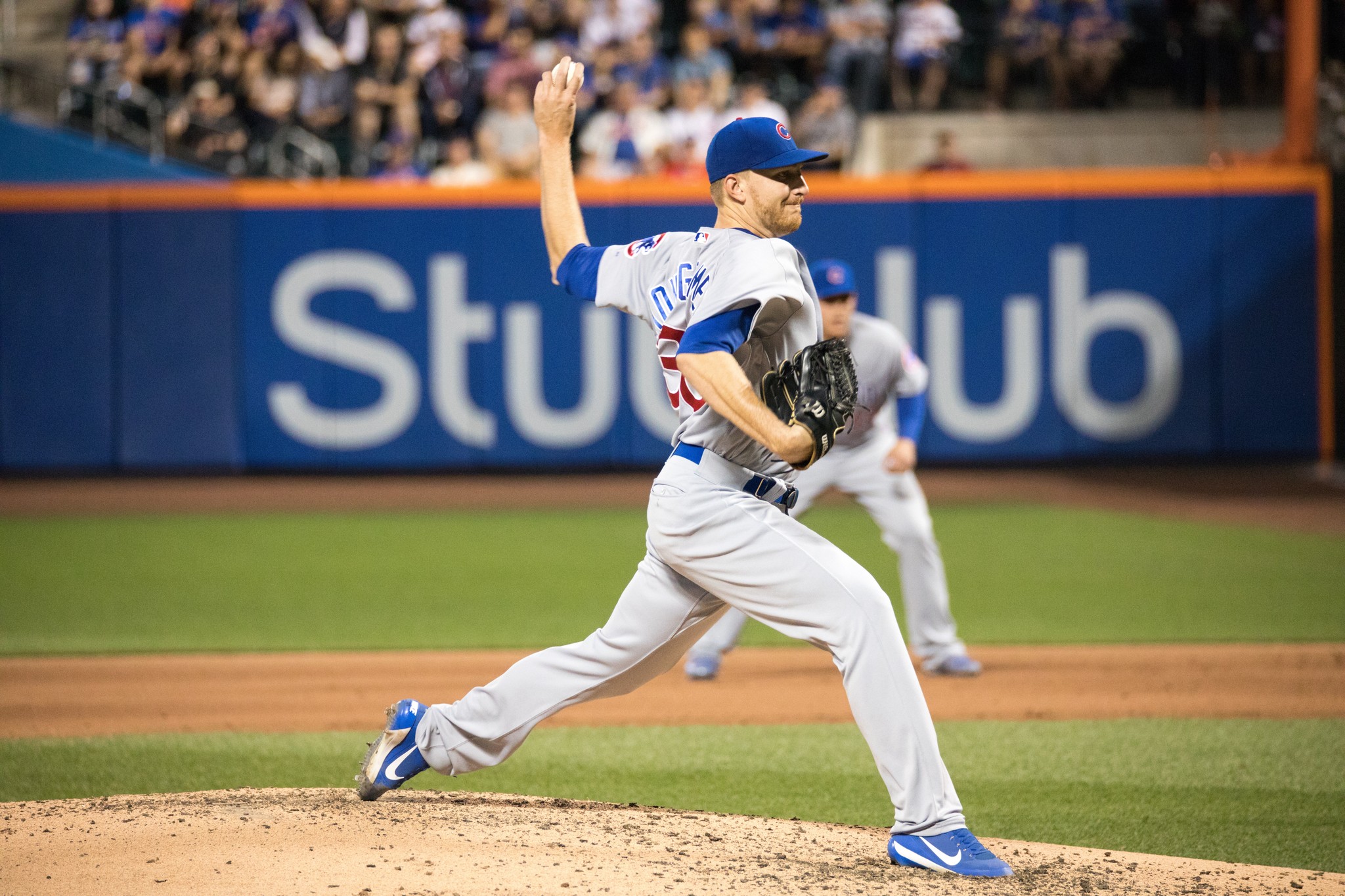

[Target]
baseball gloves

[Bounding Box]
[762,338,857,470]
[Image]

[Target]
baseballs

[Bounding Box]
[552,60,584,89]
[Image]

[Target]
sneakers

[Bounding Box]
[684,653,718,679]
[356,699,429,800]
[888,823,1015,878]
[938,651,982,678]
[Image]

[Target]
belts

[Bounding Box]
[671,443,798,510]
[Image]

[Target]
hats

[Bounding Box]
[706,116,829,185]
[807,256,854,298]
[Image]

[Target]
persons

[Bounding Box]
[354,54,1016,880]
[1,0,1345,184]
[681,258,983,677]
